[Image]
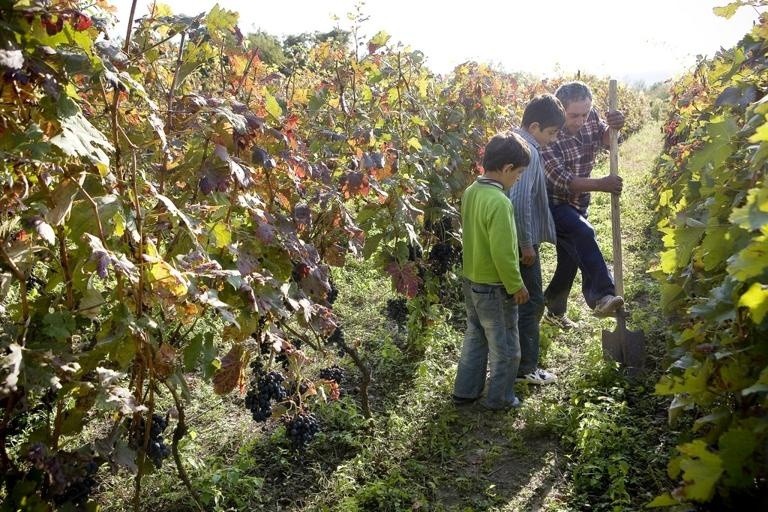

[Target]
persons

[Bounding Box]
[451,130,533,410]
[543,81,626,330]
[503,93,568,385]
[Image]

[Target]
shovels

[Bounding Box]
[602,80,644,373]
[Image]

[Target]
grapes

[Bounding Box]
[387,199,461,326]
[244,276,345,450]
[2,385,25,410]
[125,412,171,468]
[54,461,100,506]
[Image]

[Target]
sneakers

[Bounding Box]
[452,394,483,403]
[514,366,556,385]
[594,294,624,314]
[510,396,519,407]
[542,310,576,329]
